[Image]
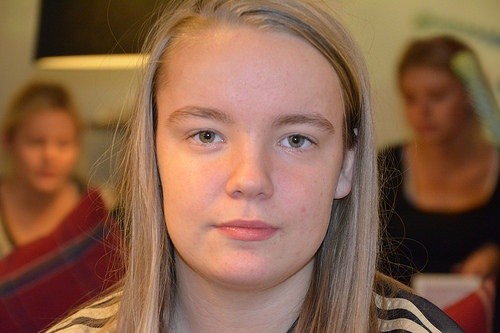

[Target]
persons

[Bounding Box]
[0,74,126,333]
[41,0,472,333]
[356,31,498,331]
